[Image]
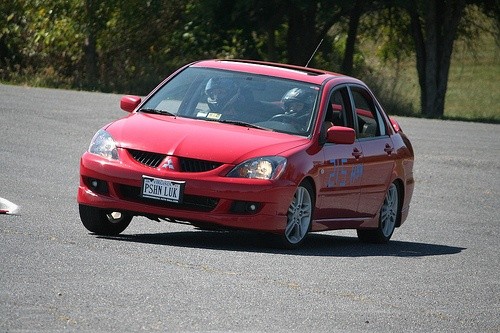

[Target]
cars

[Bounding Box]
[76,56,416,247]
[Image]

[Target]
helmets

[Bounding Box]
[281,87,315,121]
[206,77,240,112]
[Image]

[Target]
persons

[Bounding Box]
[278,87,335,134]
[197,79,248,123]
